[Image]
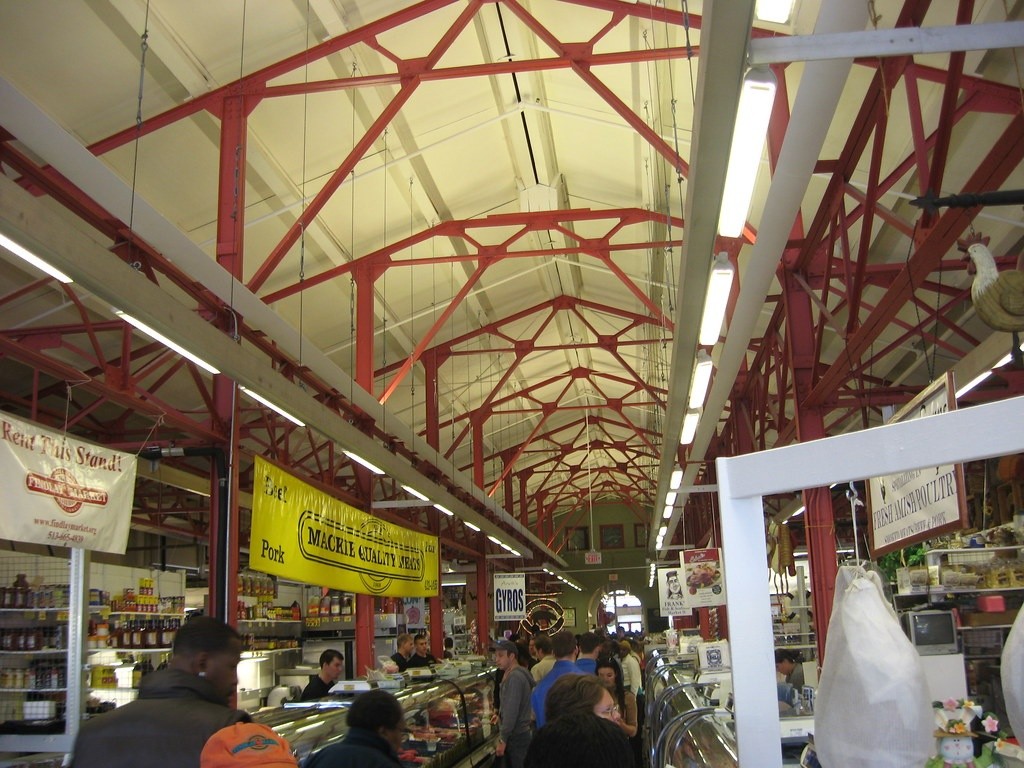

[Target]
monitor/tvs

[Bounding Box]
[899,611,958,656]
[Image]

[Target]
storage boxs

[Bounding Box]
[895,565,929,596]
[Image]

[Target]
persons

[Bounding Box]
[443,638,454,660]
[522,710,636,768]
[70,615,256,768]
[391,633,414,672]
[301,649,344,701]
[544,676,623,725]
[485,641,536,768]
[491,623,652,725]
[199,721,300,768]
[773,648,807,695]
[296,689,404,768]
[408,635,437,668]
[592,655,638,737]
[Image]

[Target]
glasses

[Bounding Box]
[594,703,621,721]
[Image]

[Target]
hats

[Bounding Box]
[488,640,519,656]
[617,640,631,650]
[200,722,299,768]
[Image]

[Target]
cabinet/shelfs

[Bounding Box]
[0,572,408,768]
[894,540,1024,710]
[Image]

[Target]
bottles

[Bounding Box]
[84,578,185,689]
[237,572,301,651]
[306,595,353,618]
[0,571,70,721]
